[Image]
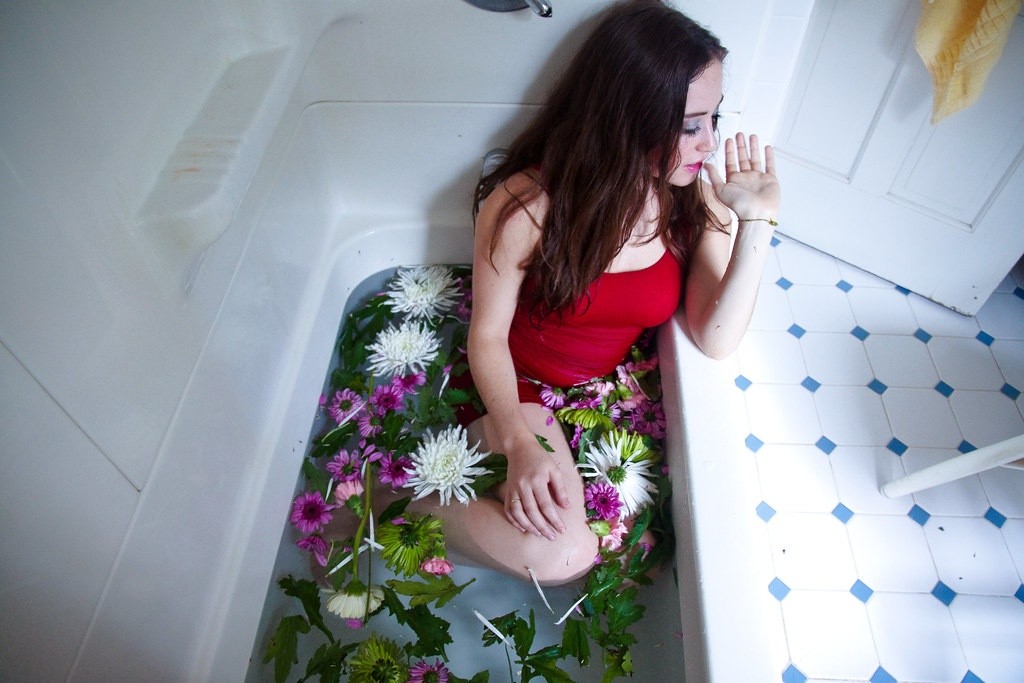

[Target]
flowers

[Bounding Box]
[325,448,363,481]
[347,630,410,683]
[540,358,667,563]
[376,512,446,578]
[384,265,464,327]
[289,491,335,534]
[364,320,445,379]
[325,460,385,619]
[368,384,405,414]
[379,451,417,489]
[357,412,384,438]
[421,530,454,575]
[402,423,495,506]
[335,479,365,519]
[345,619,363,631]
[391,371,426,395]
[295,529,328,567]
[326,387,367,423]
[407,658,449,683]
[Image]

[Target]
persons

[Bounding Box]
[318,0,779,587]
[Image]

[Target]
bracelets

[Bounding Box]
[736,217,777,228]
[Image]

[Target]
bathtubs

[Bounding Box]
[66,1,786,683]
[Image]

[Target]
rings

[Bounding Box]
[510,499,521,506]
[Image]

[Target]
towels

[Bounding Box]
[912,0,1023,126]
